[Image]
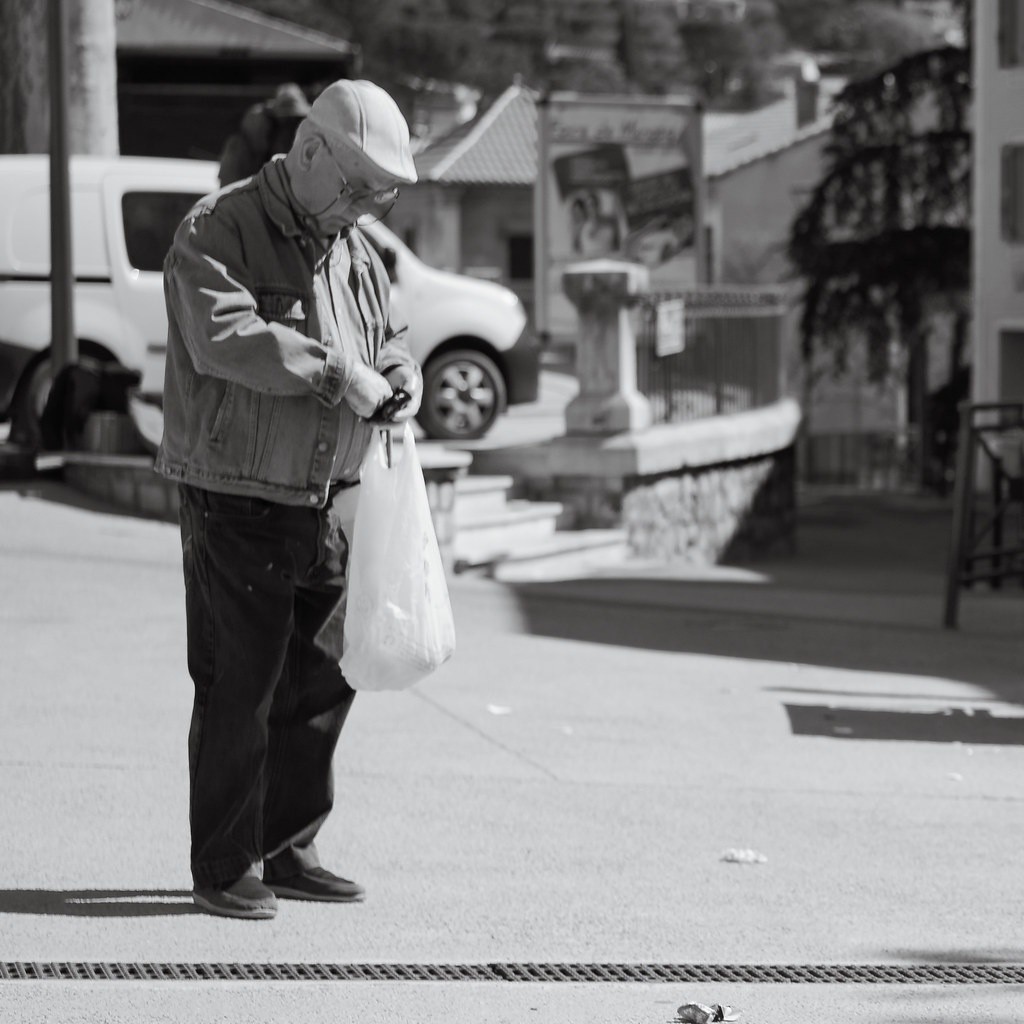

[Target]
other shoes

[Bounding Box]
[264,860,365,901]
[191,864,279,918]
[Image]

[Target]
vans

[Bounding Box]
[1,152,541,470]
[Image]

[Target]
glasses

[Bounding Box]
[310,132,399,207]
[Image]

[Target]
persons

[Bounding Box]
[155,78,424,916]
[218,83,308,188]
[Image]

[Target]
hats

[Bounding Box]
[307,78,418,184]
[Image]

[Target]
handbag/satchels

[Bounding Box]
[337,419,455,692]
